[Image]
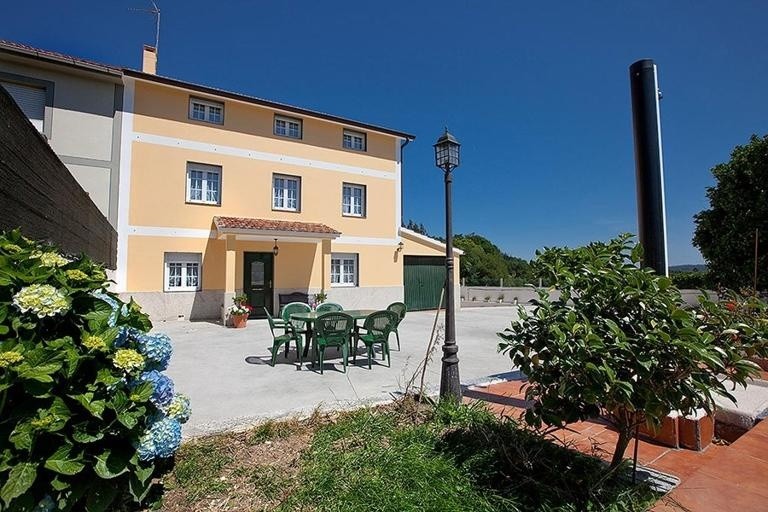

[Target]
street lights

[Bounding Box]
[433,124,463,406]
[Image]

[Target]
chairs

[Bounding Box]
[263,302,407,375]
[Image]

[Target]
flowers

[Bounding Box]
[227,293,253,318]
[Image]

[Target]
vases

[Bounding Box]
[233,315,246,328]
